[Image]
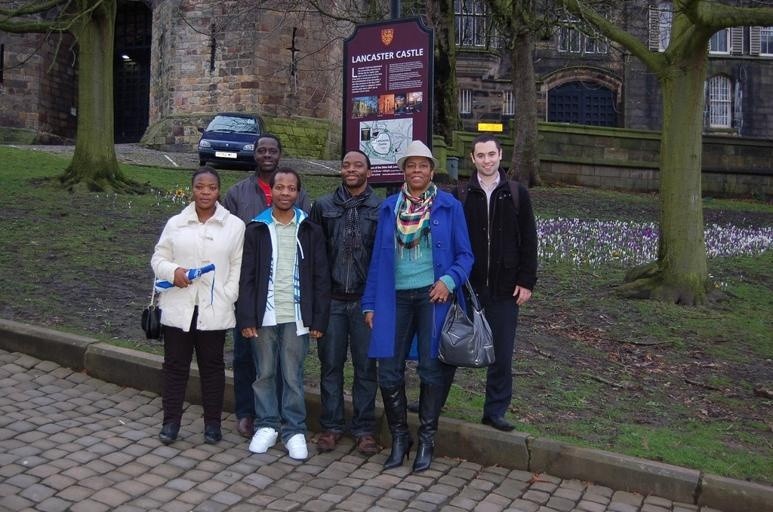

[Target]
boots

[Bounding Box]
[378,381,413,469]
[413,382,447,472]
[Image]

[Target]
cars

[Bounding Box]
[197,113,265,166]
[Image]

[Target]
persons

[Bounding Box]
[225,135,311,438]
[307,150,378,455]
[360,139,470,463]
[408,135,537,431]
[235,167,332,460]
[150,166,246,444]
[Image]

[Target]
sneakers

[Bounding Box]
[407,401,418,411]
[249,427,279,454]
[354,434,379,454]
[482,415,515,430]
[283,433,309,460]
[318,431,342,451]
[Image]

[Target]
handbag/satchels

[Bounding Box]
[438,305,495,368]
[141,304,162,340]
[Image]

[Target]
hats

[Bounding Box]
[397,139,439,171]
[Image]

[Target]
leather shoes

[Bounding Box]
[205,423,221,443]
[236,417,254,437]
[159,420,179,443]
